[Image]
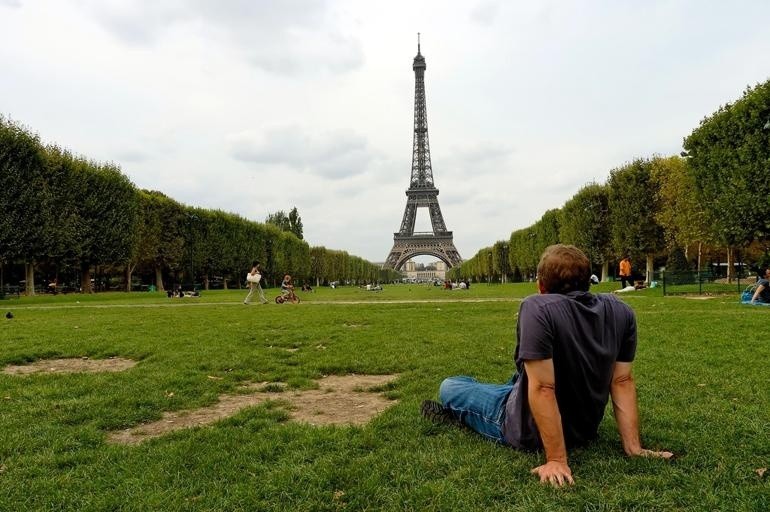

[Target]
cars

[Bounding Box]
[402,277,445,286]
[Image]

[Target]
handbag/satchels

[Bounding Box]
[246,273,262,283]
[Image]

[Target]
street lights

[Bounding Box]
[184,213,200,292]
[529,233,537,282]
[502,244,508,283]
[584,203,595,277]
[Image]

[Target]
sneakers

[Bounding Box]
[419,400,463,427]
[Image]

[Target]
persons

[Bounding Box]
[433,279,471,291]
[242,260,270,305]
[612,281,649,294]
[165,284,201,297]
[751,267,770,305]
[366,282,383,291]
[281,274,294,300]
[306,284,313,291]
[589,274,600,286]
[422,242,675,490]
[302,283,307,292]
[619,255,635,289]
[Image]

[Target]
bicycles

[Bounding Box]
[275,286,301,305]
